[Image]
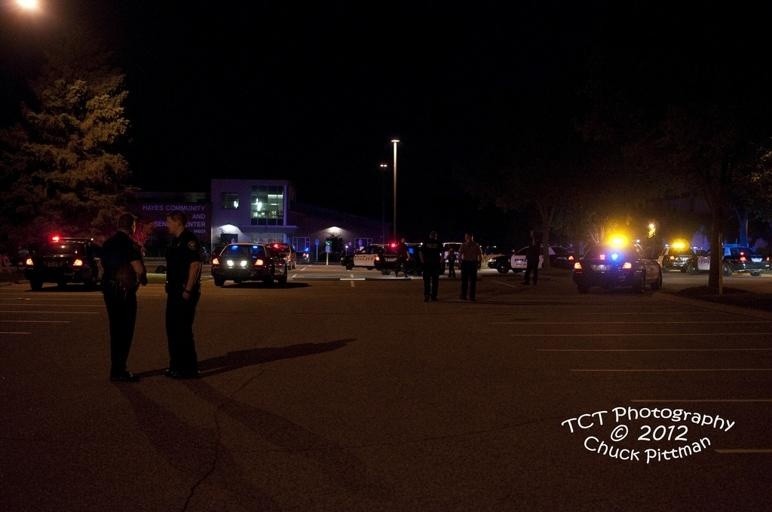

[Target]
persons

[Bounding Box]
[163,208,204,380]
[458,231,483,303]
[518,230,542,286]
[420,232,443,304]
[100,211,148,382]
[377,236,458,280]
[344,240,355,273]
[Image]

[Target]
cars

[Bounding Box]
[487,242,771,294]
[340,242,485,273]
[24,237,104,290]
[211,243,296,286]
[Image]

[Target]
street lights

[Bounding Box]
[390,139,400,240]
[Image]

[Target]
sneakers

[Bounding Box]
[423,297,478,302]
[163,364,203,379]
[110,369,140,383]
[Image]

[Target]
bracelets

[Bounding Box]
[184,288,192,295]
